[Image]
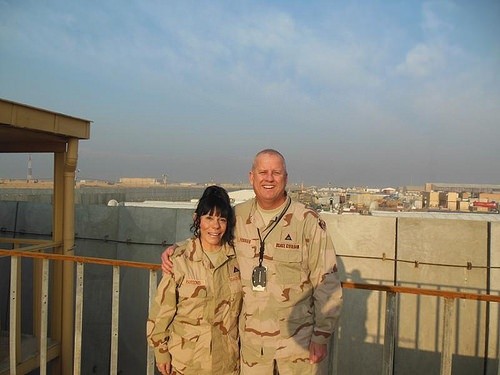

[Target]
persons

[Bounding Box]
[162,150,343,375]
[146,186,242,375]
